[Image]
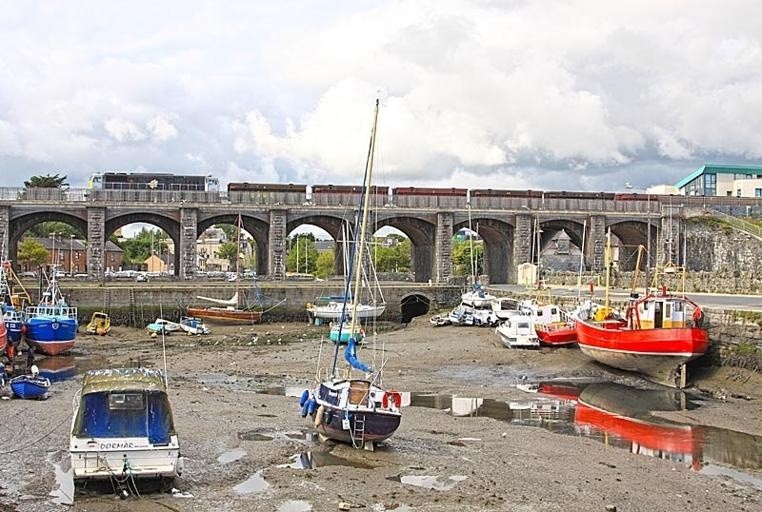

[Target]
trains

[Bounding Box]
[84,171,762,202]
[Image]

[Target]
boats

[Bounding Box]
[572,383,708,472]
[451,378,576,421]
[70,301,185,500]
[144,203,287,337]
[0,231,112,401]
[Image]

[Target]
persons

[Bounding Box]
[26,345,36,371]
[693,307,701,328]
